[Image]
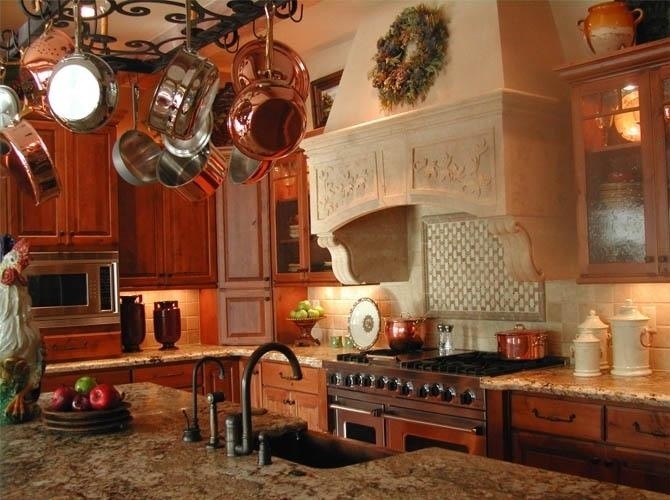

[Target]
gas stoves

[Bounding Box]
[322,347,570,411]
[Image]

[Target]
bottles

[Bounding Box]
[444,325,454,351]
[437,324,447,351]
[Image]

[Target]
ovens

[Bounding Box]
[325,387,487,457]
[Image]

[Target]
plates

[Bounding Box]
[289,226,299,238]
[43,401,133,434]
[347,296,380,351]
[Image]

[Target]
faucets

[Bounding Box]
[191,356,225,441]
[241,342,302,456]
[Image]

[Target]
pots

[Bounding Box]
[384,317,441,350]
[0,1,310,209]
[494,323,550,359]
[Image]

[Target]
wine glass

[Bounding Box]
[592,107,613,149]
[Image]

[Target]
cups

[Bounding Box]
[345,336,353,348]
[331,336,342,348]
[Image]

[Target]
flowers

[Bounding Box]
[367,4,452,112]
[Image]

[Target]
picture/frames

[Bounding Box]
[308,70,345,128]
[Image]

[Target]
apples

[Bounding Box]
[50,376,121,411]
[289,300,324,319]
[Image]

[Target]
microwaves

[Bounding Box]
[26,254,121,329]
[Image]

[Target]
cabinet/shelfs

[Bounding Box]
[39,322,121,362]
[203,357,240,403]
[118,176,218,289]
[261,360,328,433]
[269,151,340,283]
[0,120,118,252]
[131,359,203,396]
[239,357,262,409]
[199,288,309,347]
[549,36,670,283]
[40,366,132,392]
[216,146,274,289]
[511,392,670,492]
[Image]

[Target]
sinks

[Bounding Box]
[241,433,388,468]
